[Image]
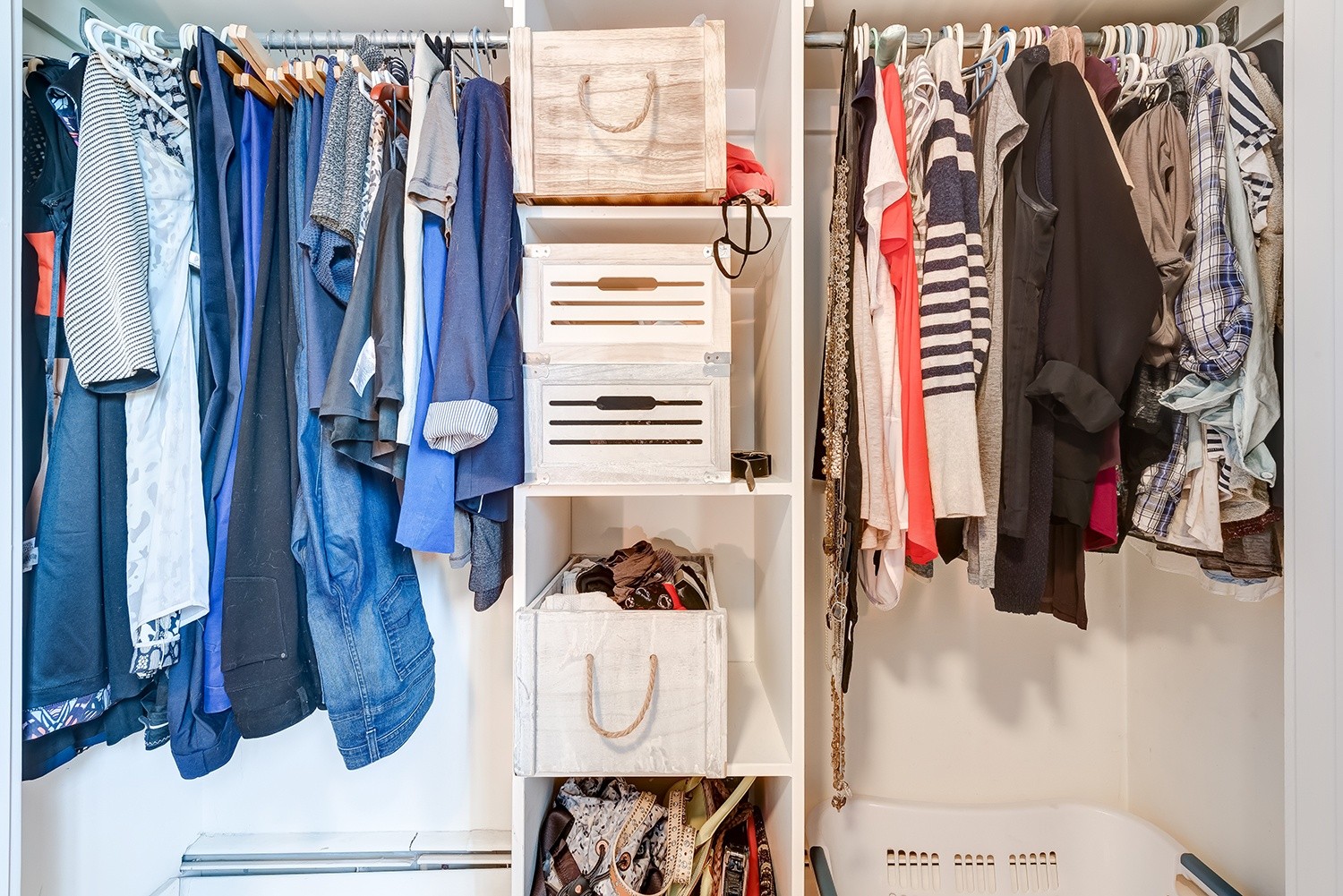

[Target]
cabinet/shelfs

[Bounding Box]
[514,0,804,896]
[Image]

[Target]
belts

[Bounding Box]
[731,450,772,490]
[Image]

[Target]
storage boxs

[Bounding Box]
[510,19,726,205]
[518,553,727,777]
[523,243,731,364]
[523,365,731,484]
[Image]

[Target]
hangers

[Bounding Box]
[1096,23,1222,111]
[836,9,1057,116]
[85,18,498,169]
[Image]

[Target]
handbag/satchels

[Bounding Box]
[712,144,776,279]
[529,776,777,896]
